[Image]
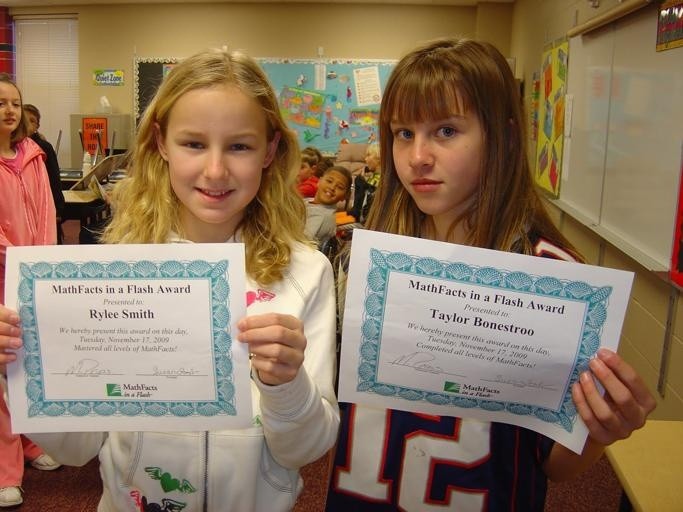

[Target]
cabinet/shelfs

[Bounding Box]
[70,113,131,170]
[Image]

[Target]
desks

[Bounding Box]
[62,190,112,230]
[60,177,85,190]
[605,419,683,512]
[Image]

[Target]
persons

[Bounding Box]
[0,74,63,506]
[324,36,658,511]
[0,41,343,511]
[22,104,66,244]
[292,141,382,271]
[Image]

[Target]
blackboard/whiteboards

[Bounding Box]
[533,0,683,293]
[133,56,400,157]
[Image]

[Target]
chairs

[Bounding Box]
[320,236,338,262]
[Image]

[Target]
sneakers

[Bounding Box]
[30,453,62,471]
[0,486,24,507]
